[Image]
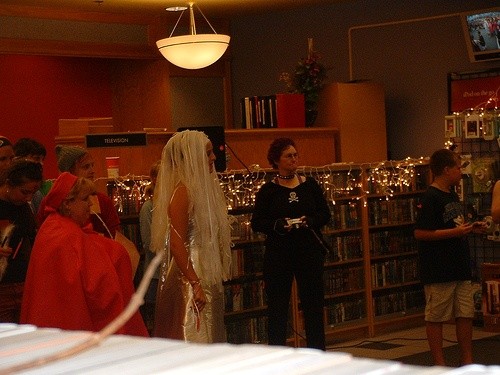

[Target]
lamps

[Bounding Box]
[156,0,230,70]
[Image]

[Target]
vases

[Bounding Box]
[305,110,318,125]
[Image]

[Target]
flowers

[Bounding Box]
[280,52,330,101]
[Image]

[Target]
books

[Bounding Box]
[322,198,424,326]
[126,223,138,247]
[223,211,268,345]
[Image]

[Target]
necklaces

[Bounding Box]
[280,175,294,179]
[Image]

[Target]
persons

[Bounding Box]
[468,16,500,51]
[19,172,149,338]
[140,159,162,328]
[55,145,121,238]
[150,130,233,343]
[414,148,489,366]
[0,137,53,285]
[491,180,500,226]
[250,138,331,352]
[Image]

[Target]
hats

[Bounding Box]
[54,144,86,172]
[35,173,79,214]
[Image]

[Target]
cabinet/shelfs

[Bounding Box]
[113,157,427,343]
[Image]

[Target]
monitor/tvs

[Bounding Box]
[460,7,500,63]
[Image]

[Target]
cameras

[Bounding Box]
[290,218,302,229]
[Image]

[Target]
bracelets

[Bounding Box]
[194,288,201,296]
[191,280,201,286]
